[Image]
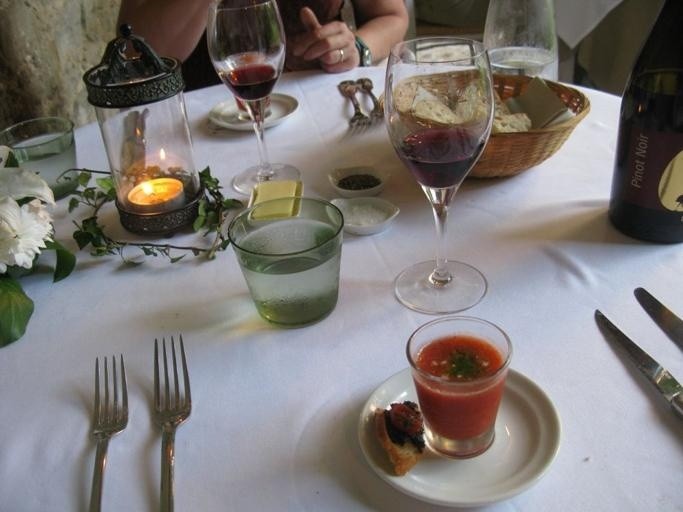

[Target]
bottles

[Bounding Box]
[605,0,682,248]
[481,0,560,85]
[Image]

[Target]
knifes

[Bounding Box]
[632,286,683,353]
[121,108,150,171]
[590,305,682,421]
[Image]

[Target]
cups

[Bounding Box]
[225,199,347,328]
[404,314,516,458]
[0,118,77,200]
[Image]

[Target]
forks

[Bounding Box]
[146,333,192,510]
[336,77,384,131]
[87,354,128,511]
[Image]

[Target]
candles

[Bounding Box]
[125,176,186,213]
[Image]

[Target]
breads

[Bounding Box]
[396,79,532,133]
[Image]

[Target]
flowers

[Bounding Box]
[0,146,80,352]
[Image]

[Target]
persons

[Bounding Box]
[116,0,409,74]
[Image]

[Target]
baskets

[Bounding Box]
[394,70,592,182]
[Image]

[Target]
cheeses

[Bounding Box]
[252,180,304,219]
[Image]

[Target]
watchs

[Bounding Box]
[354,35,372,66]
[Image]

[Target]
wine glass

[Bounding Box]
[205,1,303,196]
[383,38,496,314]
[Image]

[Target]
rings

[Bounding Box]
[339,49,344,62]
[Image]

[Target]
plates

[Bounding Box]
[353,365,563,508]
[206,91,296,131]
[327,165,386,198]
[325,196,400,235]
